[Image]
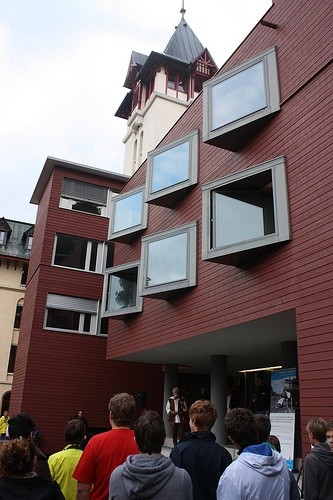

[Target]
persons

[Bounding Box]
[168,401,232,500]
[47,418,83,500]
[325,423,333,453]
[71,393,141,500]
[0,410,65,500]
[195,386,209,400]
[301,418,332,500]
[216,408,300,500]
[108,411,193,500]
[225,387,236,415]
[249,376,269,412]
[165,388,188,446]
[68,411,86,422]
